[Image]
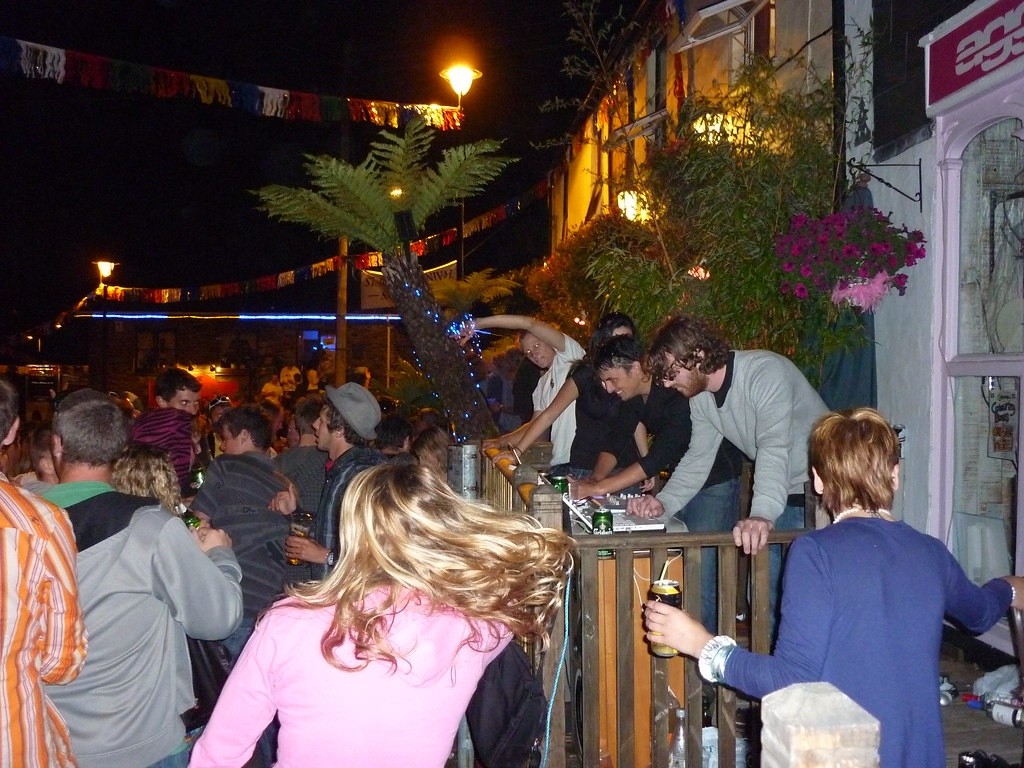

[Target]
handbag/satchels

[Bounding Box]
[669,726,748,768]
[180,633,231,732]
[466,639,547,768]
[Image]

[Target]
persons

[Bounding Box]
[567,335,745,636]
[0,314,637,768]
[626,316,830,636]
[644,405,1024,768]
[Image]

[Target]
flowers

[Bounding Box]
[774,204,927,313]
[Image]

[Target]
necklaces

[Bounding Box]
[833,508,891,524]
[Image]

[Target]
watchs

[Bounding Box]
[326,548,335,566]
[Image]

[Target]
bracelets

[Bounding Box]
[698,636,738,683]
[1010,585,1016,606]
[514,447,523,454]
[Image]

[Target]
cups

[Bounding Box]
[289,512,312,567]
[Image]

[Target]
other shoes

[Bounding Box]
[736,708,749,725]
[958,749,1020,768]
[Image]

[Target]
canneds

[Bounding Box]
[939,673,1024,729]
[649,579,683,657]
[592,508,614,560]
[190,467,205,489]
[550,476,570,502]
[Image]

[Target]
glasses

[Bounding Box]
[601,377,620,390]
[209,396,233,414]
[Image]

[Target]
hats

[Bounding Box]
[325,382,381,440]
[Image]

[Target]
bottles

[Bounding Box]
[668,710,686,768]
[986,700,1024,727]
[962,692,1024,708]
[702,697,712,728]
[178,503,201,532]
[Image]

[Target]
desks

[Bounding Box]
[530,471,687,768]
